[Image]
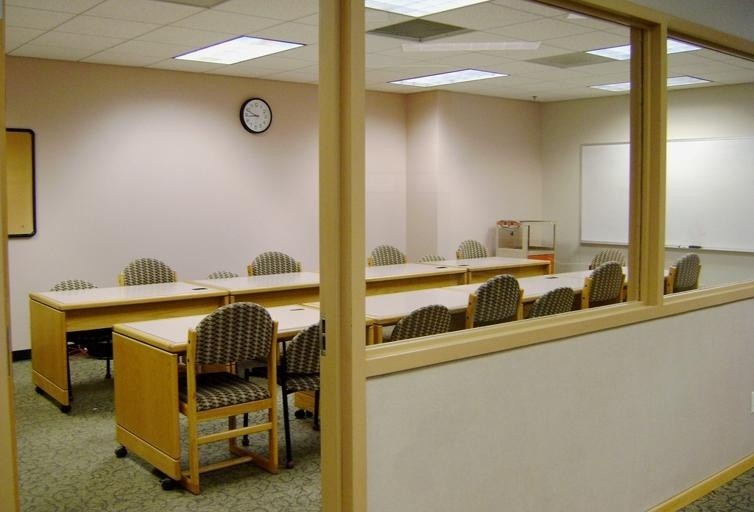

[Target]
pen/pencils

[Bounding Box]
[576,137,754,254]
[676,245,702,249]
[193,288,207,291]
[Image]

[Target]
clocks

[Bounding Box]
[238,94,272,134]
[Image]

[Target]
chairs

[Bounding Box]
[390,248,699,340]
[53,243,489,381]
[178,303,280,494]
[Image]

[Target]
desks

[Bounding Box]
[28,259,553,412]
[108,267,674,487]
